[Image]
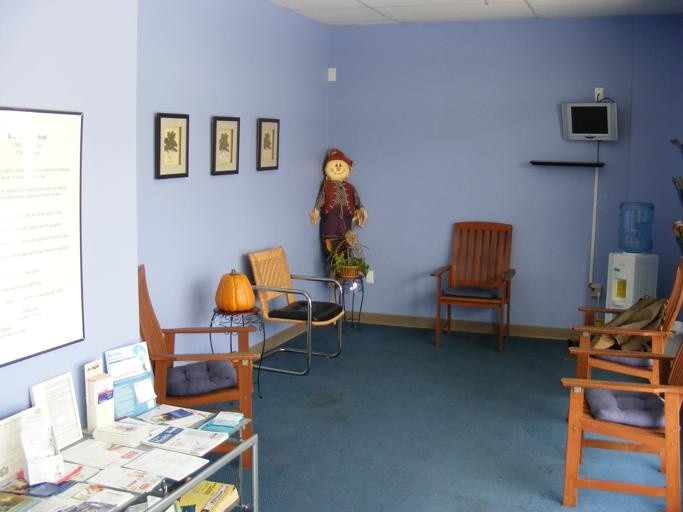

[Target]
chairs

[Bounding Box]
[248,245,346,375]
[570,257,683,397]
[559,334,683,512]
[136,264,261,470]
[429,222,517,353]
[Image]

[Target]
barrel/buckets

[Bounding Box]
[617,201,654,252]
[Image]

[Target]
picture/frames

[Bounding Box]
[211,115,241,177]
[256,118,280,171]
[156,112,189,179]
[0,106,85,371]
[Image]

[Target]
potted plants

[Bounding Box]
[332,238,370,280]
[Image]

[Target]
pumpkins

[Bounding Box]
[214,269,256,312]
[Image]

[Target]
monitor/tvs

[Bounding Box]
[561,102,618,141]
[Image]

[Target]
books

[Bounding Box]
[158,476,240,512]
[141,425,228,456]
[27,371,83,451]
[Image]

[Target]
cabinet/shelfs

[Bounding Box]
[0,402,259,511]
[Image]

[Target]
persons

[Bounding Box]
[311,149,368,262]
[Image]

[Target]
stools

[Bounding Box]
[333,274,365,330]
[209,307,265,399]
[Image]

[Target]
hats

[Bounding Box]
[324,148,353,170]
